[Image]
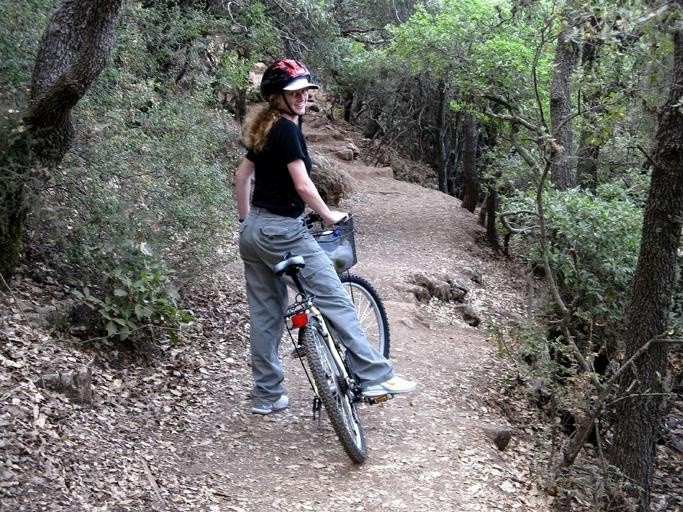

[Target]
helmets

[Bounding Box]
[260,59,310,101]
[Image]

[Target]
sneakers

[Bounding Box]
[361,375,417,396]
[251,395,289,415]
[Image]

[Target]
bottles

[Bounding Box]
[317,228,340,252]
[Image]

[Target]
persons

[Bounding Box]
[234,59,416,415]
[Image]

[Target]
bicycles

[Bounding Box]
[273,211,391,463]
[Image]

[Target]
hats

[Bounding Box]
[282,78,320,91]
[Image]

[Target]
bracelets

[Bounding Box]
[239,219,244,222]
